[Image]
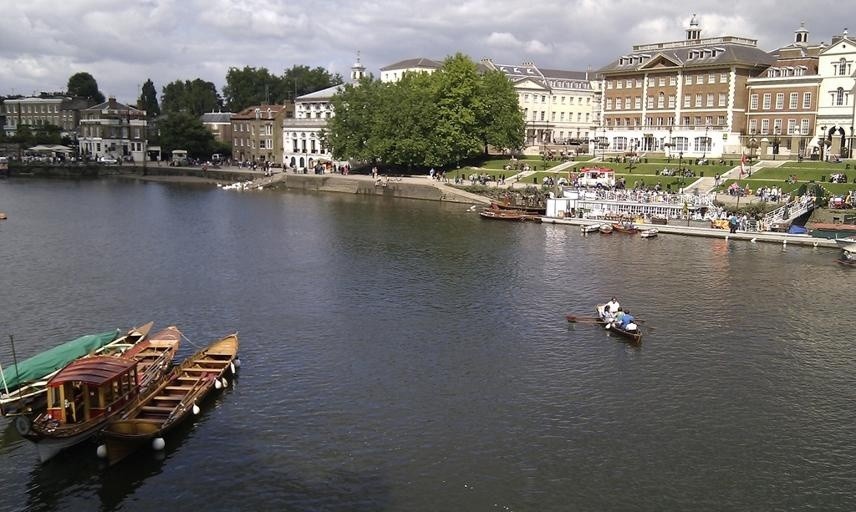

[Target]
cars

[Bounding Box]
[96,156,118,164]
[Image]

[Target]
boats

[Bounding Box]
[581,216,660,239]
[593,302,642,346]
[0,320,243,456]
[832,234,856,268]
[479,198,548,222]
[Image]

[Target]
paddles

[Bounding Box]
[163,372,214,425]
[566,316,605,324]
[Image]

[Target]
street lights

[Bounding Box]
[602,129,606,161]
[547,120,549,145]
[702,125,709,158]
[576,131,580,156]
[532,121,536,146]
[677,151,683,181]
[629,138,633,172]
[820,123,827,161]
[748,138,755,176]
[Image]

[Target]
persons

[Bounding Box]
[238,159,287,172]
[186,156,233,168]
[430,156,524,186]
[624,319,637,332]
[602,304,617,327]
[614,305,624,322]
[615,309,636,329]
[371,165,392,178]
[604,297,621,313]
[338,164,350,175]
[523,148,856,235]
[0,153,133,165]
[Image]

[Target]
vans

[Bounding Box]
[211,153,226,161]
[571,167,616,190]
